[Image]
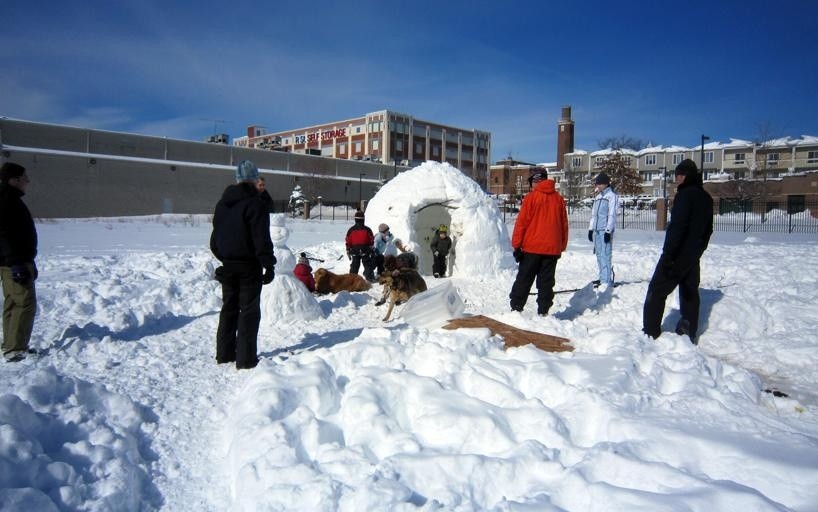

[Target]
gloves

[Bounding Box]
[513,248,522,262]
[604,232,611,243]
[262,266,275,284]
[588,230,593,241]
[11,264,31,284]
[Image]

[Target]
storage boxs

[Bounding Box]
[401,278,465,331]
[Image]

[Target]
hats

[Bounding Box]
[235,159,259,181]
[299,252,310,265]
[596,174,610,184]
[528,168,547,181]
[0,162,26,182]
[675,159,698,176]
[379,223,390,233]
[355,211,365,221]
[439,224,448,233]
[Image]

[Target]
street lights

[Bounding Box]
[359,172,366,209]
[700,134,710,182]
[657,166,667,197]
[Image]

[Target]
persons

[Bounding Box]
[293,252,316,293]
[588,173,617,289]
[0,162,38,362]
[509,167,568,317]
[430,224,452,278]
[210,159,276,372]
[345,210,418,284]
[255,177,275,215]
[640,159,714,344]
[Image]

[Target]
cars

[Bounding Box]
[613,193,655,209]
[488,191,580,209]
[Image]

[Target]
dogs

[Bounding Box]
[313,268,373,294]
[379,267,428,322]
[384,252,418,273]
